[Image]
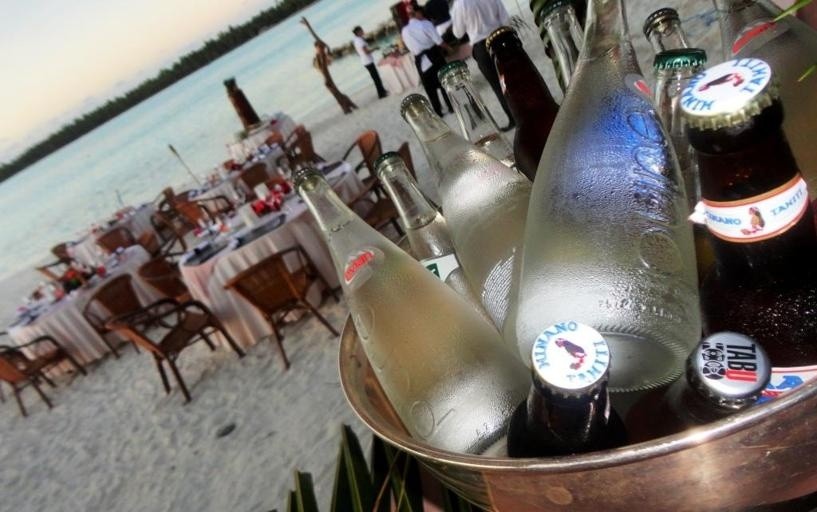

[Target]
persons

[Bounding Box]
[400,0,516,133]
[352,25,390,99]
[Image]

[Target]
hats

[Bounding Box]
[406,3,419,14]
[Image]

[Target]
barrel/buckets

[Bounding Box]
[337,204,816,512]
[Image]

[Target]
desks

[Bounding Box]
[223,113,301,176]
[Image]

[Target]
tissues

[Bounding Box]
[252,183,268,200]
[239,203,259,226]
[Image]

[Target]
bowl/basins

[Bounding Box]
[338,305,816,511]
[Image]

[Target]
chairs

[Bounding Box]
[0,126,414,420]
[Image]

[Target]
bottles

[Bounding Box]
[393,90,534,364]
[502,319,626,453]
[513,6,708,390]
[677,56,817,418]
[640,6,691,56]
[292,164,539,456]
[369,151,501,348]
[624,329,771,458]
